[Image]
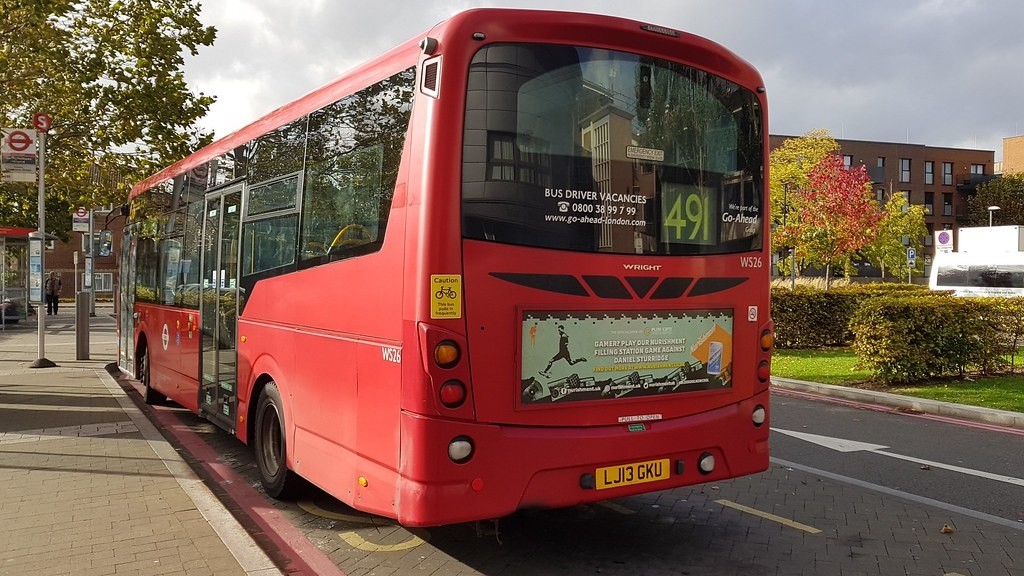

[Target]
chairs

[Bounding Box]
[305,223,373,256]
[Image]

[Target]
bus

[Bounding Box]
[98,6,775,527]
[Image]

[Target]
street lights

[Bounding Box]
[987,205,1002,228]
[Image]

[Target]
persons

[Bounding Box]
[45,271,61,315]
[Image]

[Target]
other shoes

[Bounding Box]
[54,312,58,315]
[46,313,52,315]
